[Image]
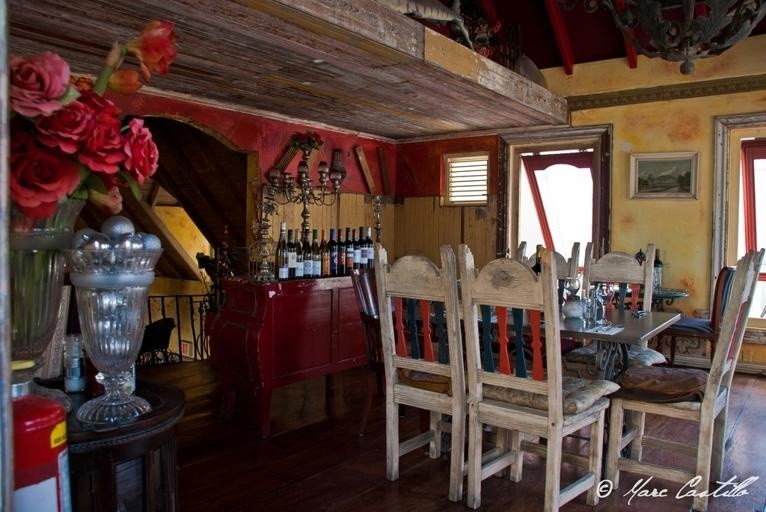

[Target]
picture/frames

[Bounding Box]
[629,151,700,201]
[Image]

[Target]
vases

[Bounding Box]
[5,194,89,375]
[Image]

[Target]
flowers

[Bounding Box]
[7,19,175,205]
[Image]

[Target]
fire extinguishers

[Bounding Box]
[13,395,71,512]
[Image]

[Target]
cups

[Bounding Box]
[581,289,596,323]
[595,283,615,327]
[563,278,579,296]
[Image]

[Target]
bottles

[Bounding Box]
[311,229,321,278]
[338,229,345,276]
[319,229,330,277]
[651,248,663,294]
[531,245,544,274]
[328,228,338,276]
[302,227,311,278]
[293,229,303,279]
[275,222,289,281]
[221,223,234,257]
[366,227,374,270]
[351,228,360,269]
[358,226,368,269]
[287,229,296,280]
[344,227,354,275]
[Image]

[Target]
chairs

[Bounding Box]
[658,265,740,374]
[604,251,765,511]
[372,241,509,503]
[567,241,655,387]
[513,241,579,301]
[457,242,601,512]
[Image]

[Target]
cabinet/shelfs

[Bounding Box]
[36,368,184,512]
[213,272,373,437]
